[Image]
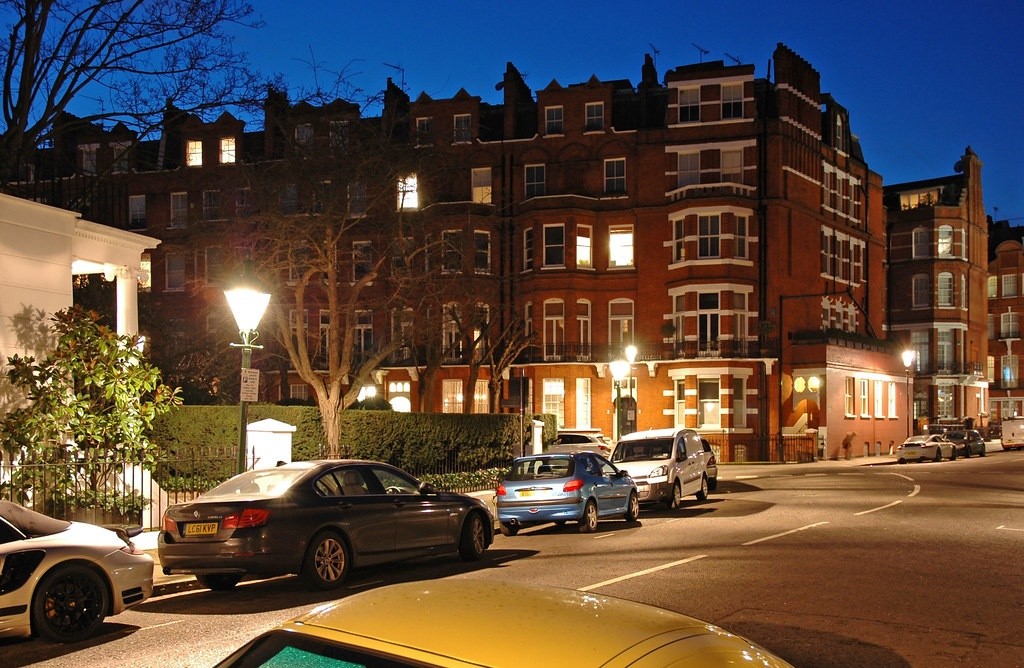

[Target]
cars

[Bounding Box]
[0,498,155,644]
[209,577,795,668]
[942,430,986,458]
[895,434,957,464]
[699,435,719,492]
[156,457,495,589]
[491,450,640,533]
[542,433,612,462]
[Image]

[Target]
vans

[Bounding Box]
[601,428,708,513]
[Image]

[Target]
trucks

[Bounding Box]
[1000,416,1024,451]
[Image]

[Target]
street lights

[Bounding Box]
[223,286,272,476]
[624,344,638,434]
[902,350,915,440]
[609,359,631,443]
[794,376,820,429]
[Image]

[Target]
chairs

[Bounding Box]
[538,464,552,474]
[339,470,367,496]
